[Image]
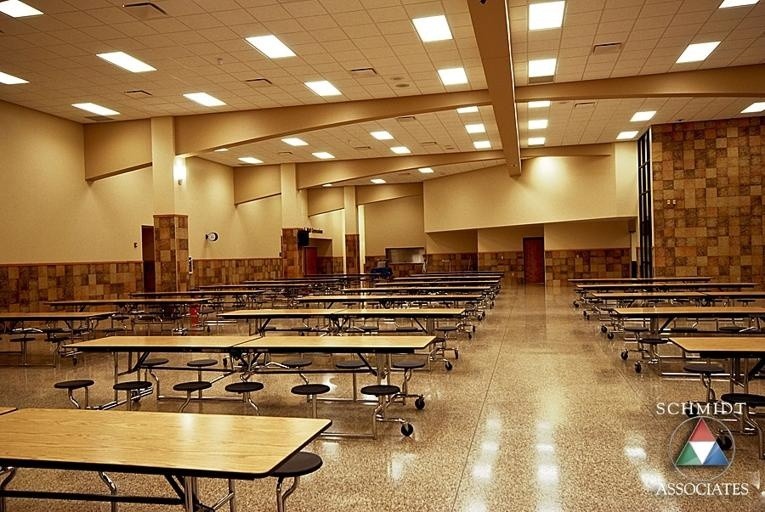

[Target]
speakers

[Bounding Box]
[297,229,309,246]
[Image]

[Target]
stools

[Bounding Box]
[568,277,765,460]
[0,273,504,512]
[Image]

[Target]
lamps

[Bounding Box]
[205,232,218,241]
